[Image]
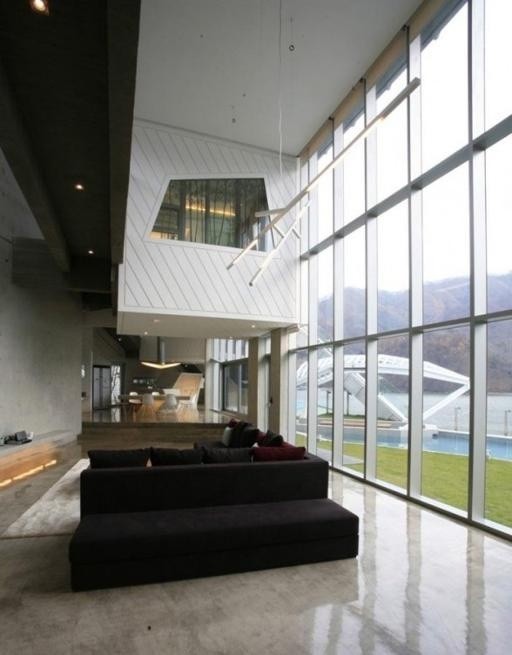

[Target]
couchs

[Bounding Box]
[68,458,358,592]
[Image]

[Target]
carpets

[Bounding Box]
[2,454,93,541]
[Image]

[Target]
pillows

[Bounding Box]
[88,418,306,469]
